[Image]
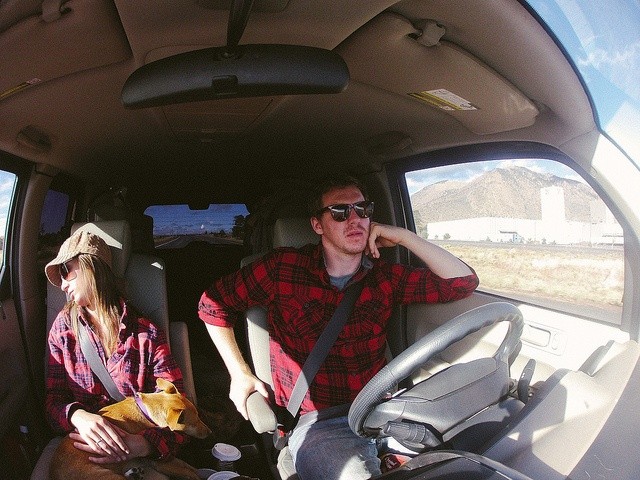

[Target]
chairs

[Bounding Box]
[238,217,322,407]
[41,219,200,409]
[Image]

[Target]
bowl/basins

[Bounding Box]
[198,469,217,480]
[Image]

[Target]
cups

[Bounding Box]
[217,458,237,472]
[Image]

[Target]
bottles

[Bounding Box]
[19,426,39,471]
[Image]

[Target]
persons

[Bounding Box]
[44,222,191,465]
[198,171,479,480]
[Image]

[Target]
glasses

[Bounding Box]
[317,200,375,222]
[56,253,81,285]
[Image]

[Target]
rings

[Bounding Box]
[96,439,104,445]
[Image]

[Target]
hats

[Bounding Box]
[45,231,111,289]
